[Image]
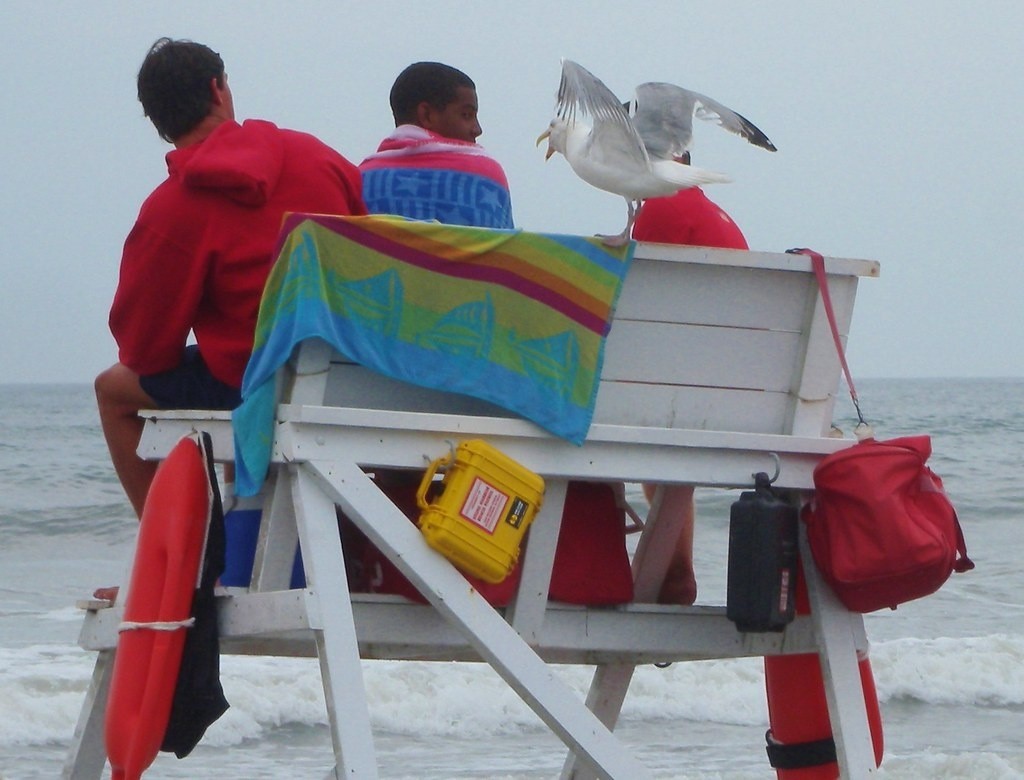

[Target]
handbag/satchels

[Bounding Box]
[801,436,974,612]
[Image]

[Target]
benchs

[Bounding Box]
[62,213,879,780]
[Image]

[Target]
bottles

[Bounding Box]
[726,472,798,632]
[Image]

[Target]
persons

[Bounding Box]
[90,36,368,607]
[632,148,751,606]
[217,61,513,595]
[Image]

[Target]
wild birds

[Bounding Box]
[536,59,777,247]
